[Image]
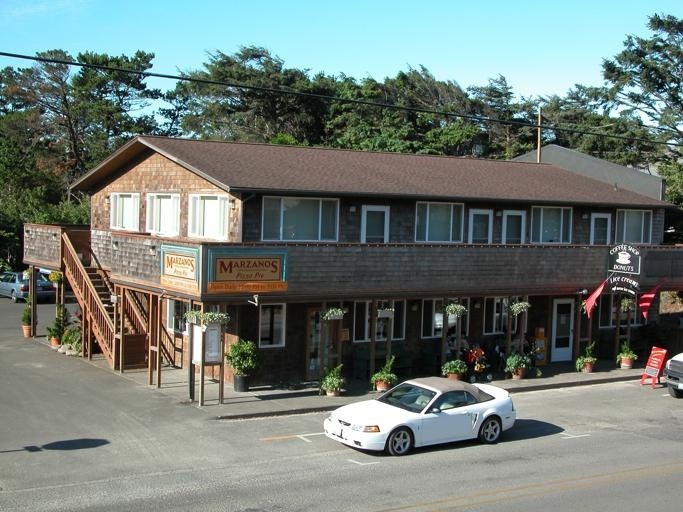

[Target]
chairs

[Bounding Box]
[439,393,458,410]
[415,389,432,407]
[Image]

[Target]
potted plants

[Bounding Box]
[225,338,257,392]
[504,352,530,379]
[320,364,344,396]
[183,310,232,325]
[576,340,597,373]
[21,295,36,338]
[321,307,345,320]
[616,341,639,369]
[377,308,394,318]
[370,354,397,392]
[46,303,67,346]
[441,359,467,380]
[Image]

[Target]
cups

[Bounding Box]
[618,252,630,262]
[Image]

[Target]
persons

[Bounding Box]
[454,331,470,360]
[445,332,457,360]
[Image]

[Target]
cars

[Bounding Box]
[322,376,516,456]
[611,299,638,324]
[22,265,61,279]
[662,352,683,399]
[366,310,393,339]
[0,270,55,303]
[434,306,456,332]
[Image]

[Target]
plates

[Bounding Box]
[616,259,631,264]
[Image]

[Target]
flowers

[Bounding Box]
[49,272,60,288]
[510,301,531,315]
[446,303,465,317]
[620,296,633,313]
